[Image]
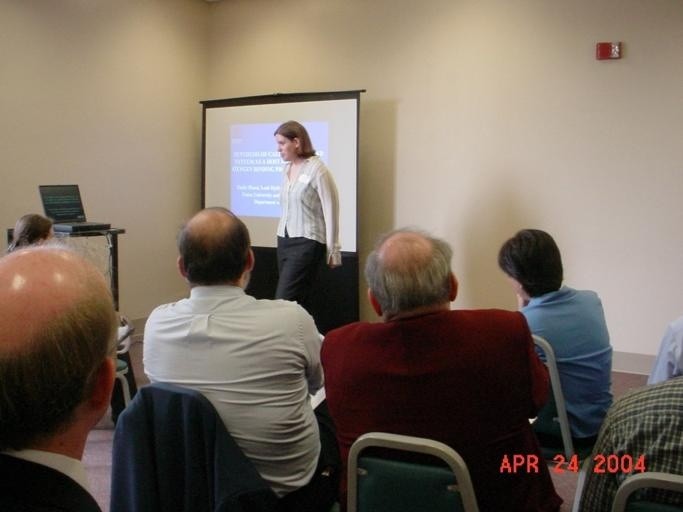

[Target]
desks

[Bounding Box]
[8,225,126,312]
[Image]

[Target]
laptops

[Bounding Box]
[37,183,111,231]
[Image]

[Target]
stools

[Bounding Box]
[115,356,132,414]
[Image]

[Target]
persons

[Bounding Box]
[579,376,683,512]
[0,238,118,512]
[142,207,340,512]
[497,229,613,460]
[274,120,342,304]
[647,316,683,385]
[7,213,137,428]
[320,227,564,512]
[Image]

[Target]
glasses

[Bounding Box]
[87,313,135,382]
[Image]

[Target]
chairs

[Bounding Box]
[346,432,478,512]
[612,472,683,512]
[531,335,596,512]
